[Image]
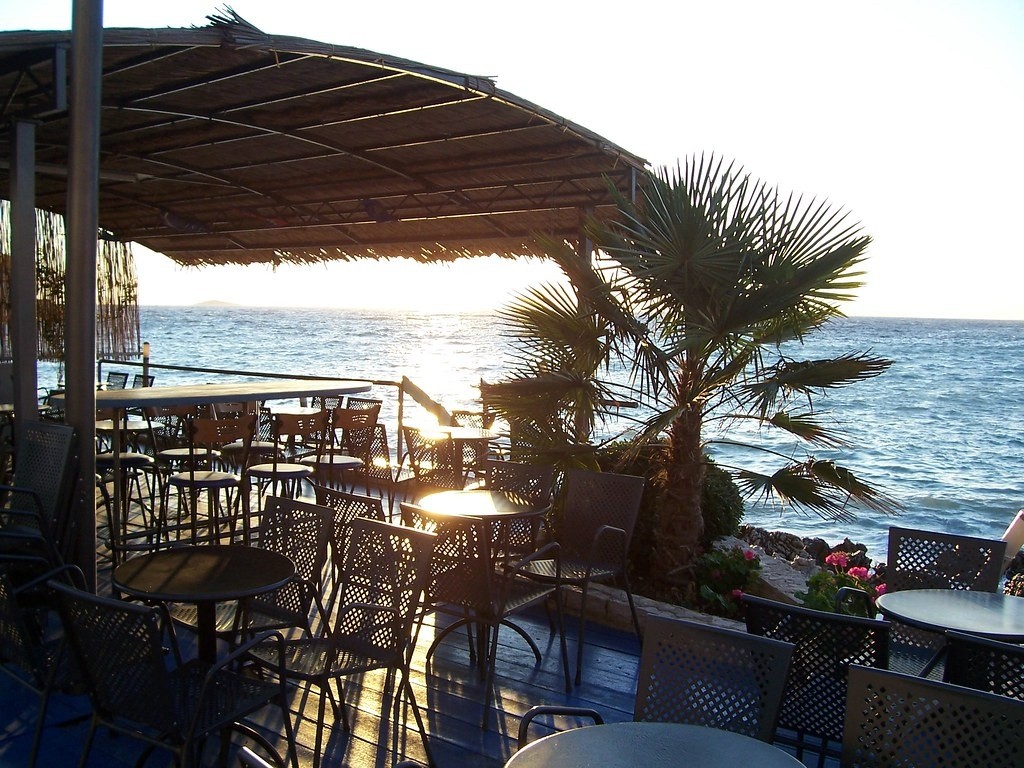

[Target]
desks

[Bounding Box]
[50,380,371,545]
[110,544,297,768]
[505,721,805,768]
[414,488,551,665]
[873,590,1024,696]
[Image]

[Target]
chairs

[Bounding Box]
[0,370,1024,768]
